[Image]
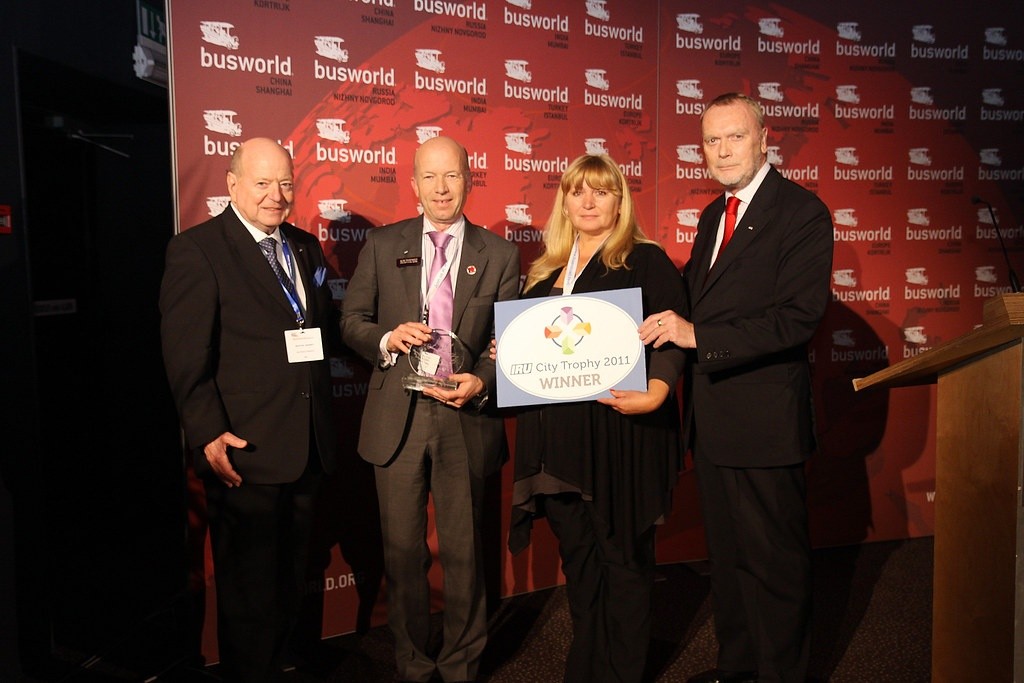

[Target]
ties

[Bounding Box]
[419,231,454,382]
[258,237,305,323]
[717,197,741,261]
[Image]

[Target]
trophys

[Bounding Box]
[401,328,465,391]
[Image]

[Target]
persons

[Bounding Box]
[340,135,521,682]
[489,153,692,682]
[639,91,834,683]
[156,136,354,682]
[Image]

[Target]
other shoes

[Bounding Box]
[685,668,760,683]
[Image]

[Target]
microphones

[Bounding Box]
[972,195,1024,294]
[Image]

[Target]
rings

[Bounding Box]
[658,320,661,326]
